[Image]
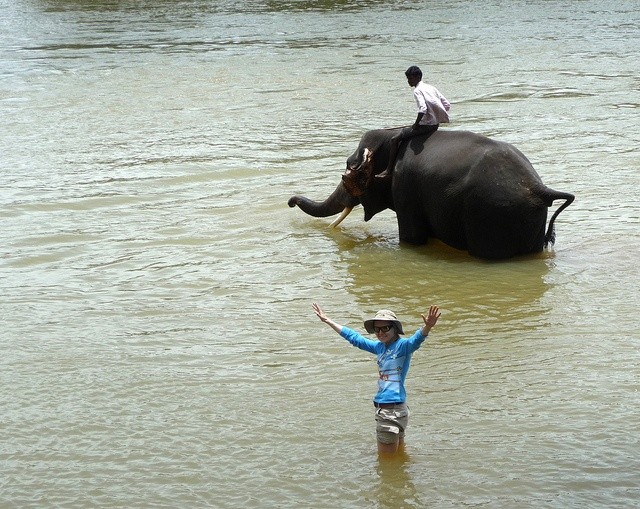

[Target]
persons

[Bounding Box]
[374,66,451,180]
[310,301,442,466]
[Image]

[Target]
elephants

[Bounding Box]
[285,125,578,263]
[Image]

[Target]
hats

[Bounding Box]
[364,310,405,336]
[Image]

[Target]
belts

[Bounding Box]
[374,402,401,408]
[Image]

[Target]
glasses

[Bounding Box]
[373,324,394,332]
